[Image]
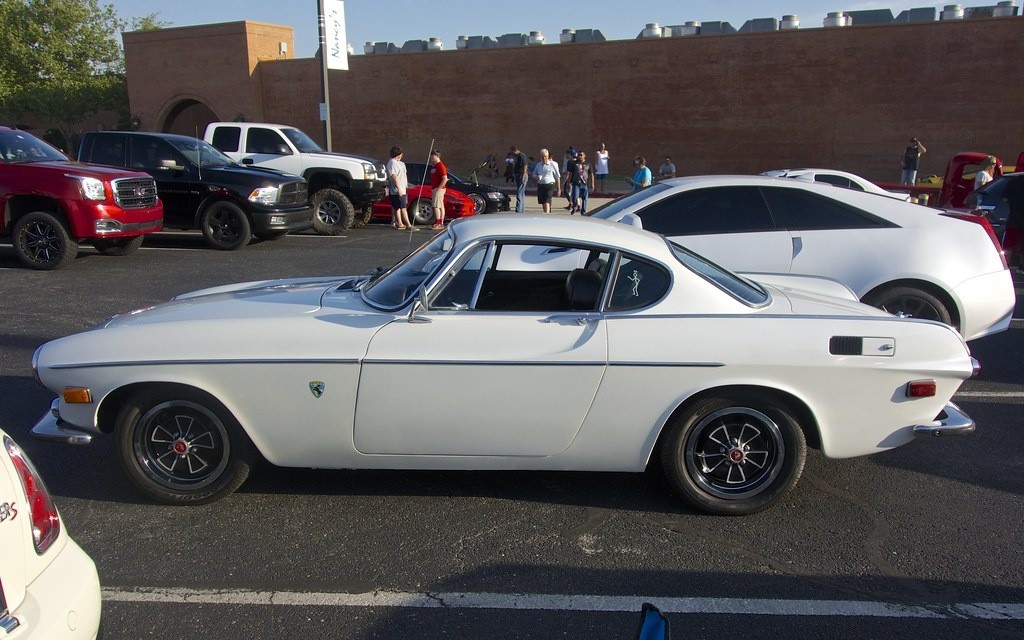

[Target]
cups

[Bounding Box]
[538,175,542,180]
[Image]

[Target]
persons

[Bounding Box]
[594,142,610,191]
[562,146,594,216]
[386,146,420,231]
[429,149,448,230]
[532,149,561,213]
[503,152,554,188]
[899,137,926,186]
[512,145,528,213]
[974,156,997,190]
[626,156,652,191]
[656,157,676,182]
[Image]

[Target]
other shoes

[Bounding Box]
[398,225,407,230]
[406,226,420,232]
[576,205,581,212]
[564,203,571,209]
[430,224,445,230]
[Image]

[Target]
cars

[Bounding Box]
[421,174,1017,347]
[942,174,1024,268]
[0,125,163,271]
[774,168,912,202]
[1,427,102,640]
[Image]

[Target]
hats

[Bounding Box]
[911,138,918,142]
[570,151,578,158]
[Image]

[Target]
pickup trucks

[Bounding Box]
[72,131,315,252]
[869,152,1005,208]
[203,121,390,235]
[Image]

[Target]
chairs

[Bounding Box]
[589,259,609,279]
[248,134,275,153]
[132,144,171,168]
[566,268,604,310]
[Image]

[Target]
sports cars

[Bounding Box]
[371,182,474,226]
[402,153,512,215]
[30,212,979,516]
